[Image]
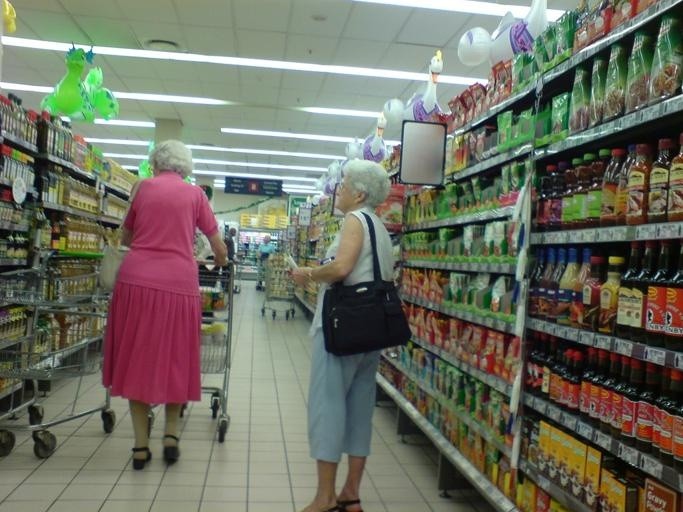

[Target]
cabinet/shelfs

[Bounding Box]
[286,195,343,314]
[377,82,516,512]
[233,228,282,283]
[515,1,682,512]
[0,130,131,401]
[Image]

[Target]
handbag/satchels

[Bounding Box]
[96,234,141,293]
[321,276,413,358]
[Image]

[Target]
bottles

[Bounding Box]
[524,333,681,476]
[532,136,683,232]
[1,92,138,393]
[524,239,682,353]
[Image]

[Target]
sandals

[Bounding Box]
[337,498,364,512]
[131,445,154,470]
[324,504,337,512]
[162,434,181,463]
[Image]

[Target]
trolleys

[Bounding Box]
[0,284,116,460]
[256,257,270,293]
[261,256,297,320]
[223,257,244,294]
[144,258,234,443]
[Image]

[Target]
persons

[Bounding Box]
[102,139,229,469]
[291,158,394,512]
[256,235,275,290]
[194,184,224,325]
[221,228,239,293]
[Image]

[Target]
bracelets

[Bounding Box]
[304,268,316,282]
